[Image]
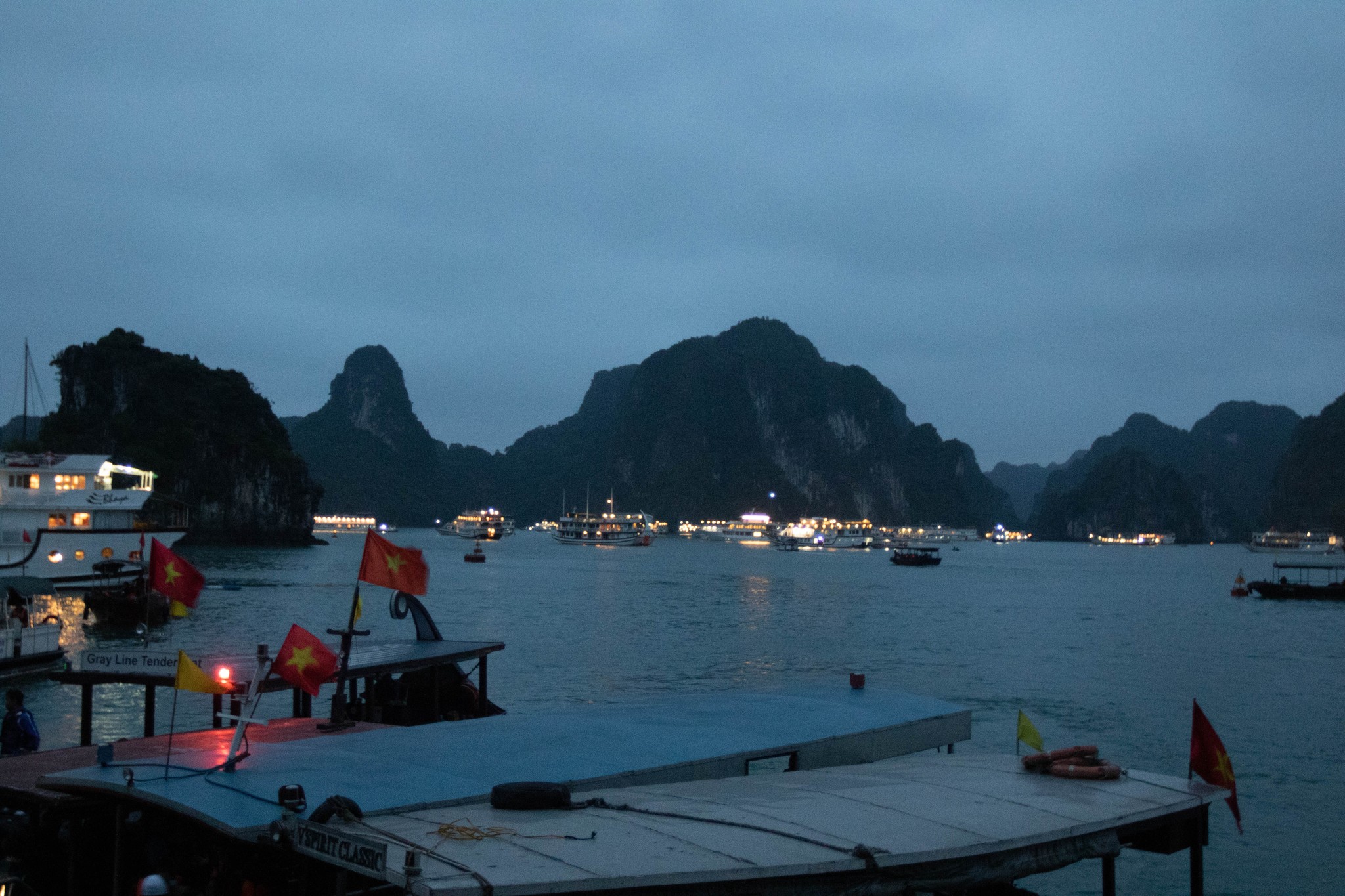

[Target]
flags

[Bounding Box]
[174,650,227,694]
[357,527,430,596]
[270,623,338,697]
[149,536,205,609]
[353,596,362,625]
[1190,698,1243,834]
[1017,709,1043,752]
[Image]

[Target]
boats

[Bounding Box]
[892,547,943,566]
[1241,526,1343,555]
[313,513,398,534]
[1246,560,1345,600]
[551,481,657,547]
[464,540,486,563]
[0,612,1245,896]
[525,509,1176,551]
[1,336,186,597]
[434,509,515,540]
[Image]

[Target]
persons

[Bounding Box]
[0,688,40,805]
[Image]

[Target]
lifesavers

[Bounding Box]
[1020,744,1099,769]
[1049,755,1123,780]
